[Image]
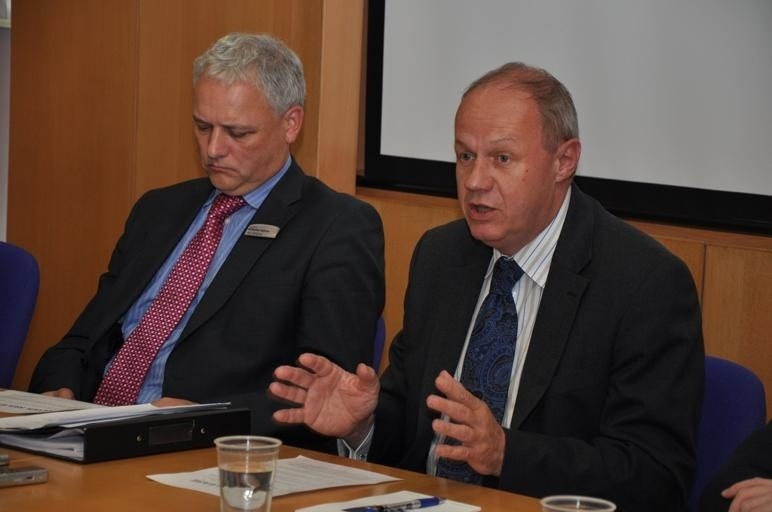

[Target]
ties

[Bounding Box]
[92,191,248,405]
[432,257,525,487]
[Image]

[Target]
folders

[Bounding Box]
[1,407,252,464]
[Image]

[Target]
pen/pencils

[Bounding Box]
[343,496,448,512]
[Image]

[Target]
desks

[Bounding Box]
[5,383,627,511]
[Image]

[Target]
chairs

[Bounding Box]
[2,243,48,388]
[688,345,766,508]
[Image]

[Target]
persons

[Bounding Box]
[696,416,772,510]
[23,31,389,460]
[266,57,708,511]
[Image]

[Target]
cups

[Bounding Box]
[215,435,282,510]
[540,495,617,512]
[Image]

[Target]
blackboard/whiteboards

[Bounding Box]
[365,2,772,235]
